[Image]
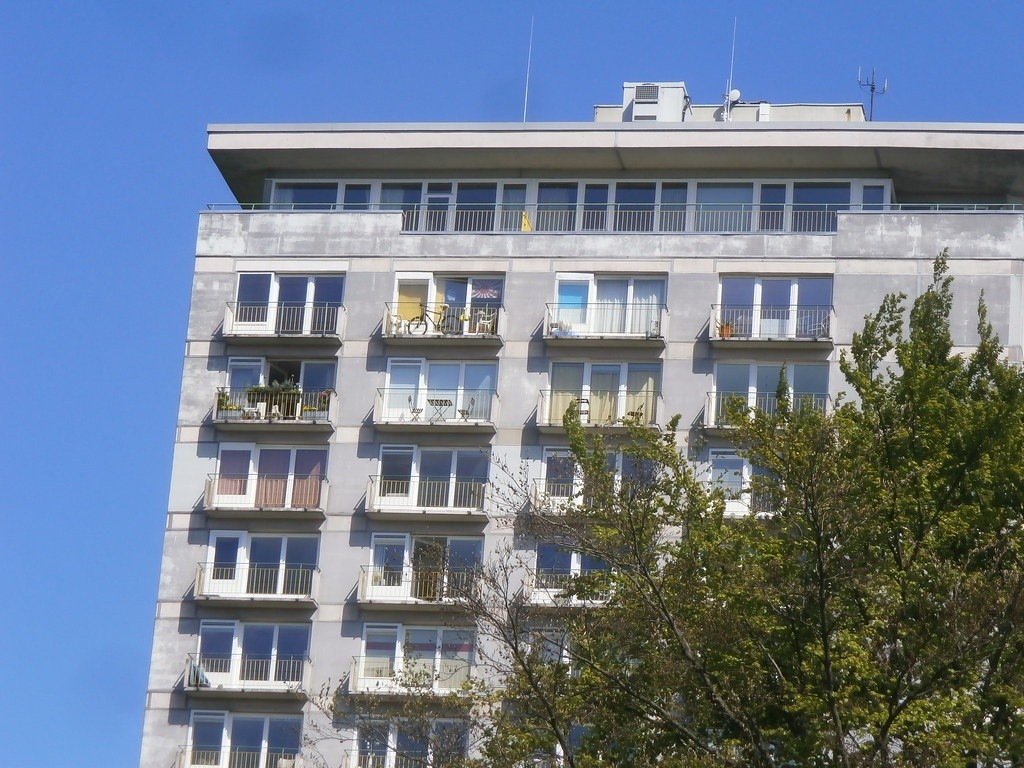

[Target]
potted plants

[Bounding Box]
[715,315,743,338]
[246,382,299,404]
[241,412,248,419]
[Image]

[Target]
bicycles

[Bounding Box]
[408,302,461,335]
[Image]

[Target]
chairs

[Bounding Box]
[407,394,423,423]
[277,758,295,768]
[456,396,476,421]
[474,313,498,336]
[576,398,591,422]
[627,401,645,422]
[387,308,411,334]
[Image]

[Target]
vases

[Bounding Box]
[217,410,243,419]
[303,411,328,420]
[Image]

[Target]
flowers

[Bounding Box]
[218,390,242,411]
[304,388,338,411]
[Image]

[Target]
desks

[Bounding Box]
[427,399,453,422]
